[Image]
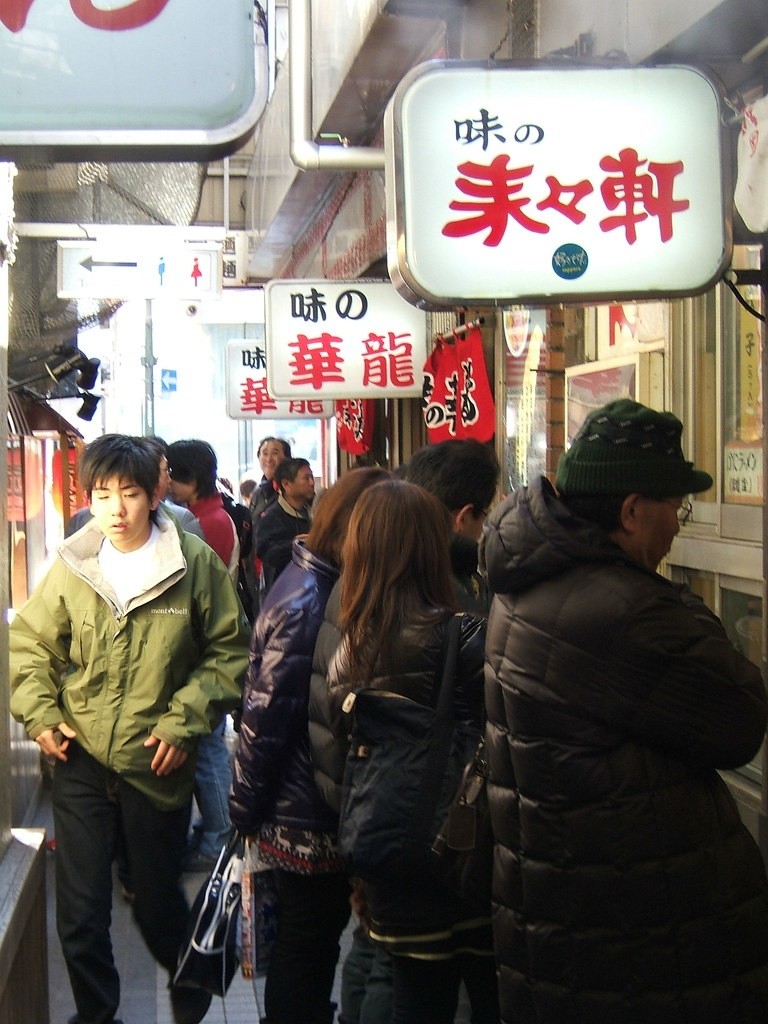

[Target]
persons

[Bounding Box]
[8,434,253,1024]
[256,459,315,595]
[227,467,392,1024]
[239,478,258,503]
[476,397,768,1024]
[65,439,240,901]
[308,436,501,815]
[249,437,292,516]
[325,479,490,1024]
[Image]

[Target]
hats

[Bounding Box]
[555,398,713,497]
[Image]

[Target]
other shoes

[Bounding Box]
[181,846,231,874]
[172,828,244,999]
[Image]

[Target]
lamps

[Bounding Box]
[8,345,101,421]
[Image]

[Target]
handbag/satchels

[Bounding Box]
[233,833,271,978]
[429,731,498,884]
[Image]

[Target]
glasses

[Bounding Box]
[157,465,172,477]
[662,499,692,527]
[479,507,491,519]
